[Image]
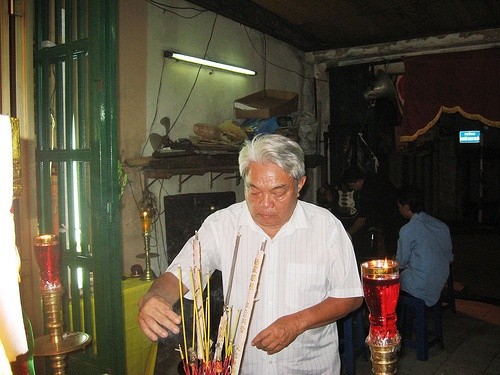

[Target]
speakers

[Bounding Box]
[163,191,236,267]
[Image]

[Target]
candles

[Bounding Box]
[32,235,61,290]
[360,261,401,343]
[139,210,151,233]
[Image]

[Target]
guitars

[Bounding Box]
[334,135,357,220]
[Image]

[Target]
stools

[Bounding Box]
[398,295,444,360]
[336,312,367,375]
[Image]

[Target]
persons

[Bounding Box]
[335,170,455,306]
[138,132,367,374]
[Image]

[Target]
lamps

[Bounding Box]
[163,50,257,78]
